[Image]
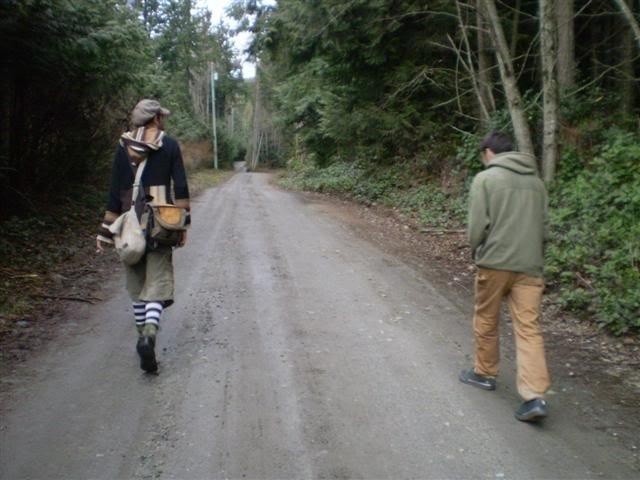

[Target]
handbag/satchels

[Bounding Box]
[148,204,187,246]
[110,208,146,265]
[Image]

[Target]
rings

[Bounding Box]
[96,250,100,253]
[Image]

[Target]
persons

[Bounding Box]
[96,99,191,376]
[458,132,550,420]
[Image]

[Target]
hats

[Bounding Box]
[132,99,170,125]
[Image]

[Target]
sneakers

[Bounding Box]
[137,336,157,371]
[515,399,547,423]
[460,370,495,390]
